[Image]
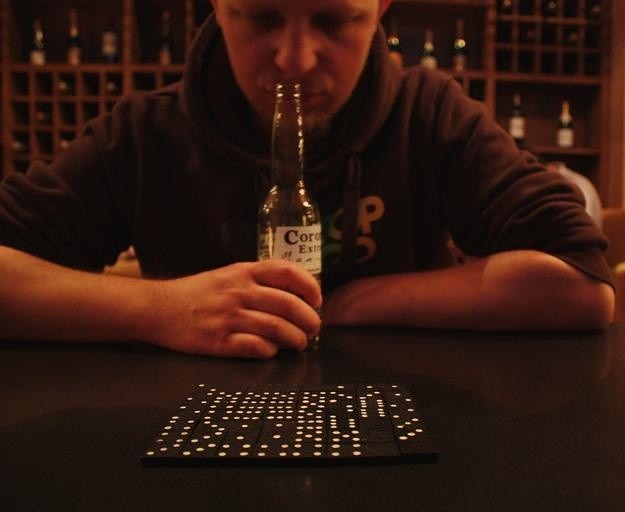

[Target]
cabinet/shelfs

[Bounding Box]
[0,0,612,209]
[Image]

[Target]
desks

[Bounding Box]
[2,316,624,510]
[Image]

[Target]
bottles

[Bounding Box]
[251,83,325,340]
[11,6,177,68]
[56,82,74,95]
[501,0,512,15]
[36,111,50,123]
[11,141,28,153]
[507,91,528,146]
[383,11,469,70]
[554,101,574,148]
[567,33,578,45]
[545,2,556,17]
[106,82,120,95]
[589,5,602,19]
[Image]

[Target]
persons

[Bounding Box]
[0,0,616,359]
